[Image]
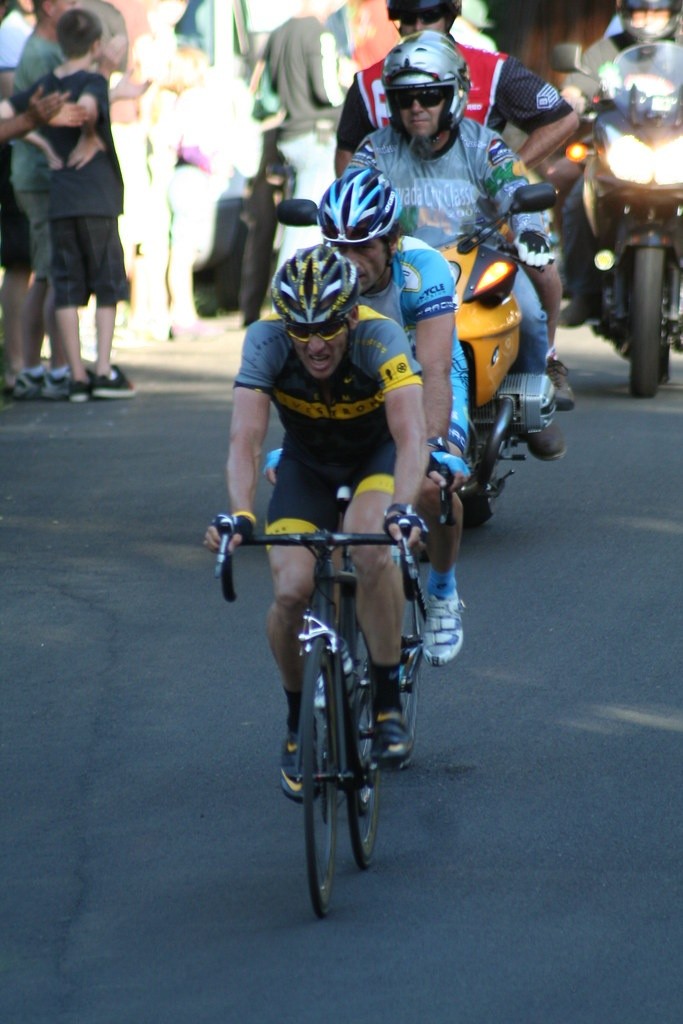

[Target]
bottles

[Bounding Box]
[336,641,358,693]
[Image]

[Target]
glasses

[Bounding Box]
[386,87,444,110]
[285,319,346,341]
[395,7,443,24]
[327,239,372,254]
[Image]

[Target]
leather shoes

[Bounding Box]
[529,424,566,462]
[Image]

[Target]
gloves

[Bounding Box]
[514,231,556,267]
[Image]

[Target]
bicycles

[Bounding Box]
[212,435,469,923]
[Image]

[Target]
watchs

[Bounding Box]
[428,436,449,453]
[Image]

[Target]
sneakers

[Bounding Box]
[42,371,72,400]
[91,364,137,399]
[370,708,412,770]
[424,590,467,665]
[69,368,96,402]
[12,372,42,399]
[547,355,575,411]
[279,735,320,803]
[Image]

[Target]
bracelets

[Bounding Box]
[232,511,256,523]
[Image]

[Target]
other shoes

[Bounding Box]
[559,300,599,329]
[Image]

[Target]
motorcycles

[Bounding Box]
[552,35,683,403]
[274,181,565,529]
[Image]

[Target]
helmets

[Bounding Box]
[382,29,471,128]
[319,167,402,243]
[272,244,360,323]
[387,0,462,31]
[624,0,682,18]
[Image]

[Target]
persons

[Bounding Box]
[163,48,234,341]
[561,0,683,328]
[0,0,154,403]
[262,1,345,201]
[345,29,566,461]
[333,0,580,412]
[263,163,469,667]
[201,245,429,801]
